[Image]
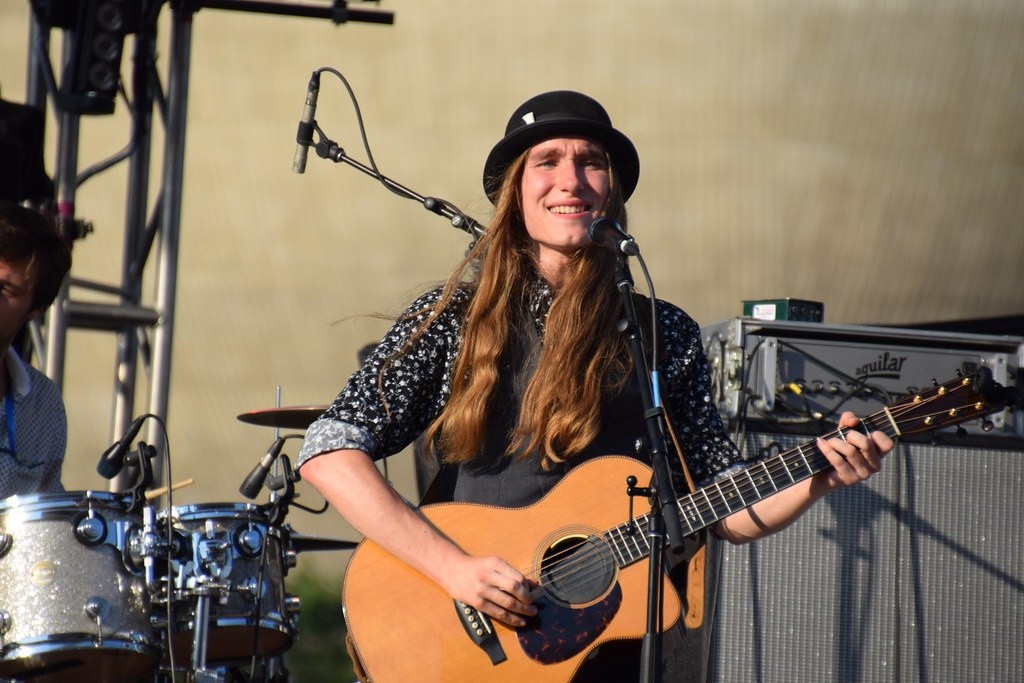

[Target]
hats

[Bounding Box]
[484,90,640,204]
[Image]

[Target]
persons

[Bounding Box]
[291,91,898,683]
[1,200,71,497]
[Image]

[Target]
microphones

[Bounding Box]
[589,216,638,257]
[240,436,285,500]
[292,71,321,174]
[97,414,147,479]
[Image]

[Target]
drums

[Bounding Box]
[151,503,302,667]
[0,489,151,682]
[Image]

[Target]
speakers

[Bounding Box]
[706,420,1024,683]
[60,0,131,115]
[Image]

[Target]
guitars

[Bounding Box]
[335,362,1005,683]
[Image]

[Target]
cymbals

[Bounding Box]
[289,533,360,553]
[235,403,334,429]
